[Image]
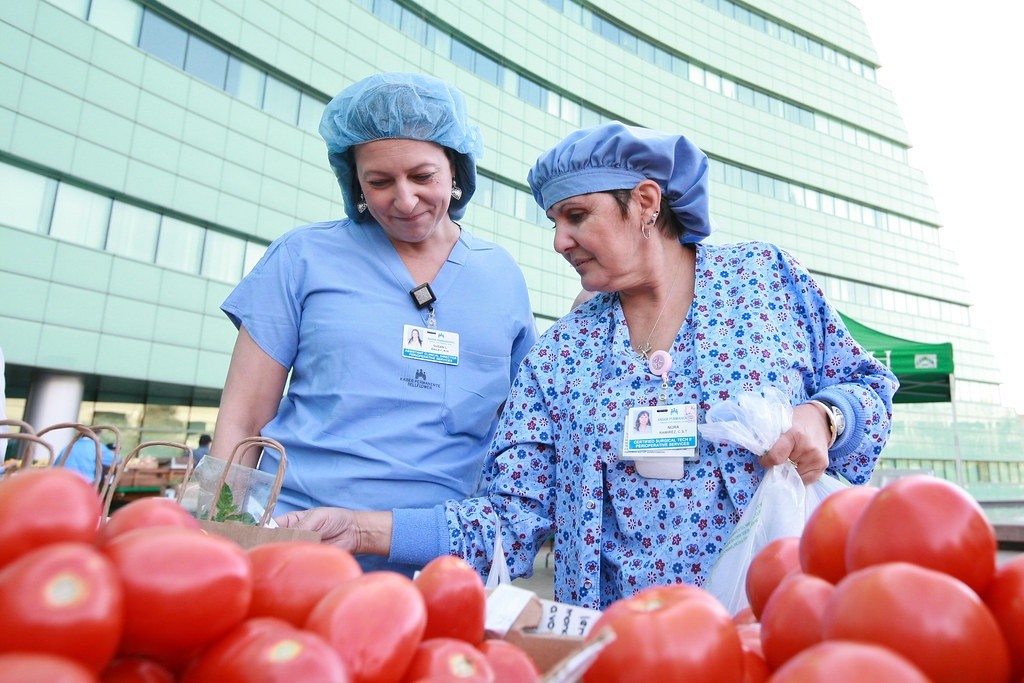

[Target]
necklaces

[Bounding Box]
[625,251,684,360]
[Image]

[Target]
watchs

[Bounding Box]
[821,401,846,440]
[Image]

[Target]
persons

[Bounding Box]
[408,329,422,347]
[53,426,122,488]
[632,411,653,434]
[210,70,542,581]
[277,119,900,612]
[187,435,213,467]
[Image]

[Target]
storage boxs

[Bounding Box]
[98,459,188,487]
[485,583,583,674]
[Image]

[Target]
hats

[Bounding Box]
[528,120,712,244]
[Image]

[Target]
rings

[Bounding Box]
[787,458,798,468]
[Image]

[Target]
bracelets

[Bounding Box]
[803,399,837,450]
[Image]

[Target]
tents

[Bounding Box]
[835,310,964,485]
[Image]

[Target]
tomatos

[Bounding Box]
[0,464,1024,683]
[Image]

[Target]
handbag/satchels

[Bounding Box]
[192,436,321,552]
